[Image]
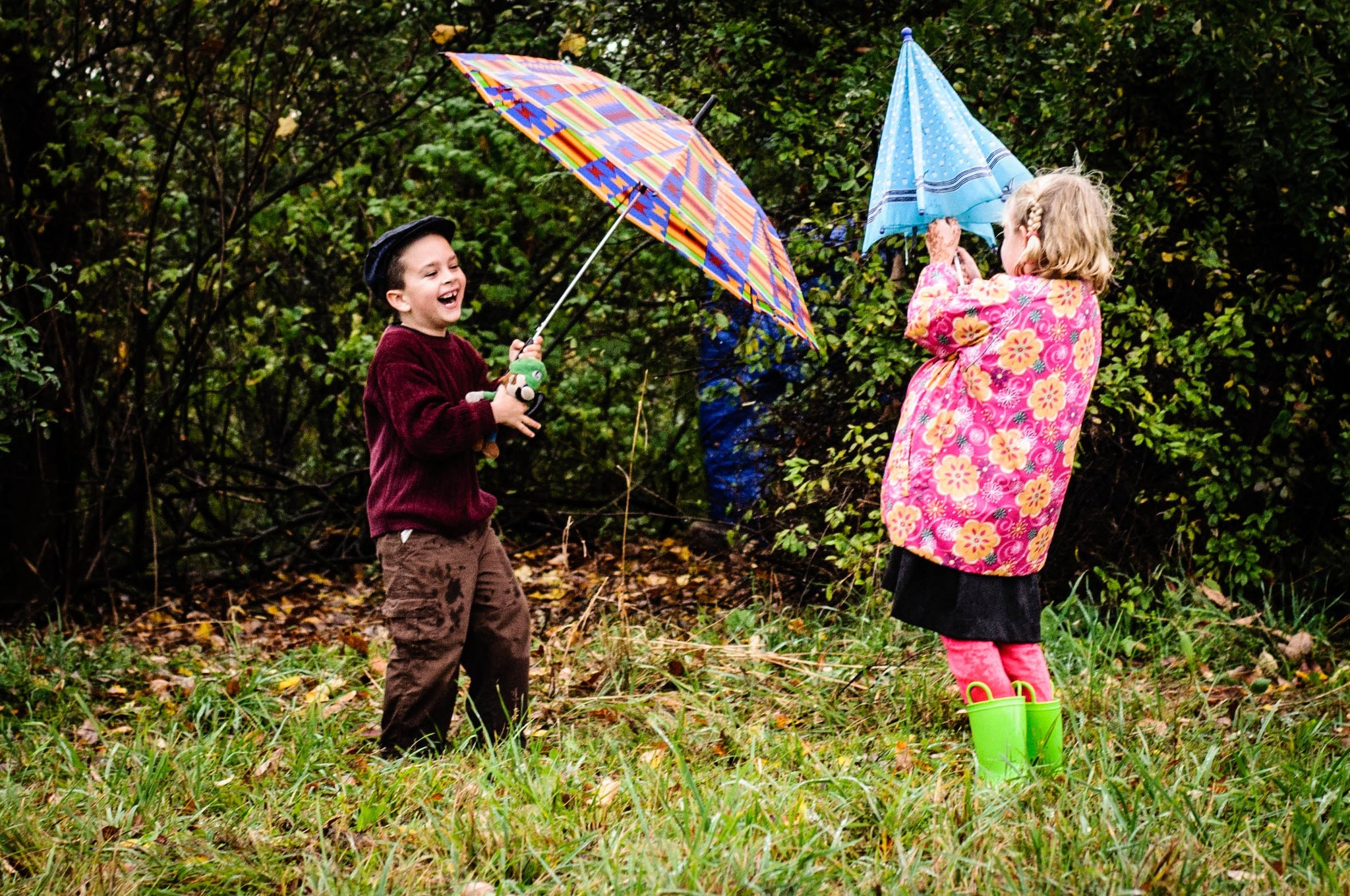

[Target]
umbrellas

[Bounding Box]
[862,25,1036,291]
[444,44,821,361]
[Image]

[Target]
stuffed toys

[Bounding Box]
[466,353,548,466]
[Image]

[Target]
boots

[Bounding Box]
[1014,681,1064,773]
[965,681,1029,797]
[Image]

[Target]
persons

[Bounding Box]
[361,215,535,759]
[878,171,1115,780]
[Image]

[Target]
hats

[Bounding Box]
[363,215,457,300]
